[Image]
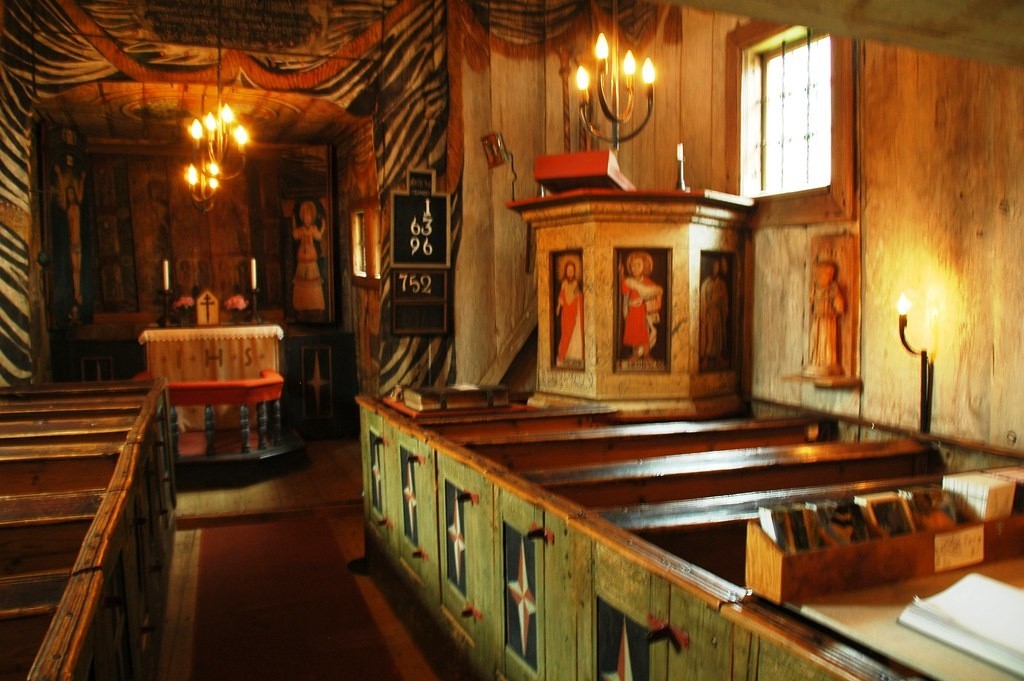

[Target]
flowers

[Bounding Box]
[223,294,249,310]
[173,295,195,309]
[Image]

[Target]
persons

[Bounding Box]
[807,260,844,369]
[292,202,328,311]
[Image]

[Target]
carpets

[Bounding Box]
[188,508,404,680]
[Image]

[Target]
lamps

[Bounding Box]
[180,0,252,212]
[573,0,658,149]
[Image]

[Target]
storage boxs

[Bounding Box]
[745,477,1024,603]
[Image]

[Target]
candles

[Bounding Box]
[251,257,257,289]
[163,258,170,290]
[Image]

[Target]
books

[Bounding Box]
[896,572,1024,681]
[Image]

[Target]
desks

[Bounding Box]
[0,377,178,681]
[353,389,1024,680]
[139,323,284,433]
